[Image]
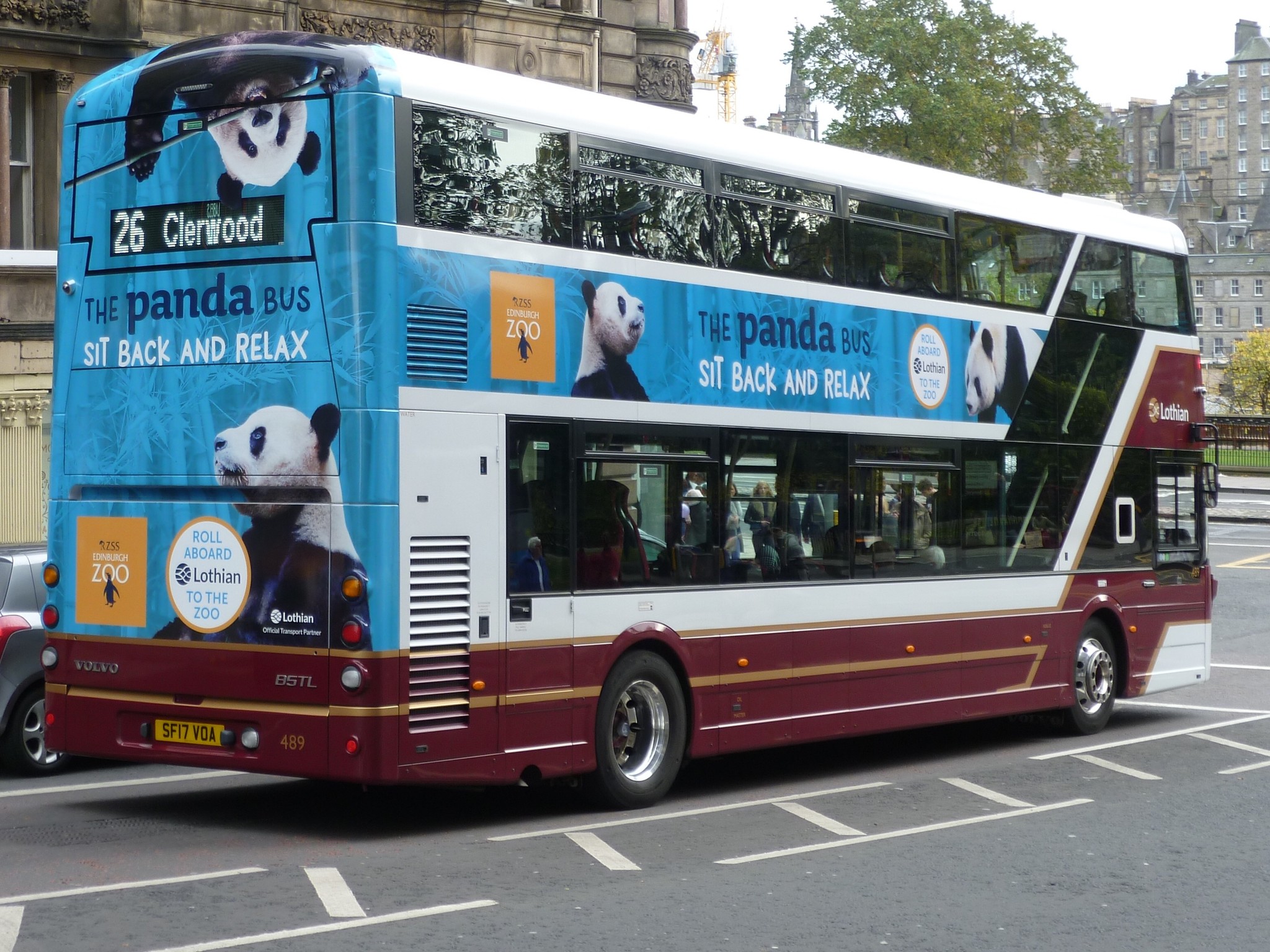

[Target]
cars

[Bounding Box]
[0,543,78,778]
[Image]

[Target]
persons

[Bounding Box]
[868,262,892,288]
[680,471,708,547]
[869,541,897,579]
[912,261,940,290]
[578,487,624,591]
[922,545,946,576]
[511,534,553,593]
[770,526,810,581]
[873,471,939,552]
[656,532,696,585]
[1031,512,1056,533]
[744,482,776,557]
[726,483,744,559]
[800,494,843,558]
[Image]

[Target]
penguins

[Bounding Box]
[517,329,533,363]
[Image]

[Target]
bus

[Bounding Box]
[40,27,1225,813]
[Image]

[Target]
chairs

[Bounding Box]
[585,220,946,294]
[507,480,934,593]
